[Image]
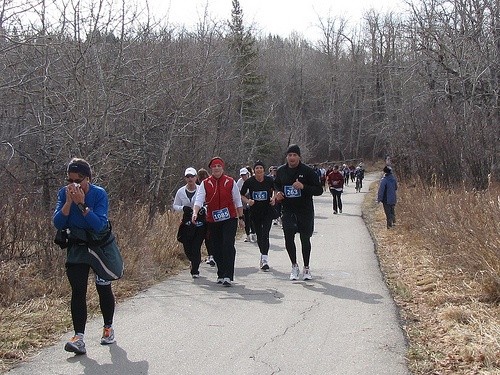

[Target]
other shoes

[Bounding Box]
[217,278,224,283]
[244,237,251,242]
[303,266,312,280]
[251,233,256,242]
[261,264,269,271]
[206,259,215,266]
[290,264,300,280]
[223,278,231,286]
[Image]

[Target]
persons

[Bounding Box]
[310,163,365,193]
[236,167,257,243]
[173,168,208,278]
[377,166,397,229]
[267,166,283,225]
[195,168,210,185]
[276,144,324,280]
[245,166,254,178]
[240,162,279,271]
[192,157,245,287]
[52,158,115,354]
[326,166,344,214]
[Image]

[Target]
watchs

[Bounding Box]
[77,203,89,213]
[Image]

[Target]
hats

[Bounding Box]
[288,144,301,156]
[239,168,248,176]
[383,166,391,173]
[185,168,197,177]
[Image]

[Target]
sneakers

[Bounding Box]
[65,340,87,355]
[100,326,115,345]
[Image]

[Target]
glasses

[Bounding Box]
[68,177,85,183]
[186,175,196,178]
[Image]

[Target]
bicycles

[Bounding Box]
[353,176,363,193]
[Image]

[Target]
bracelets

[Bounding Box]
[239,214,245,221]
